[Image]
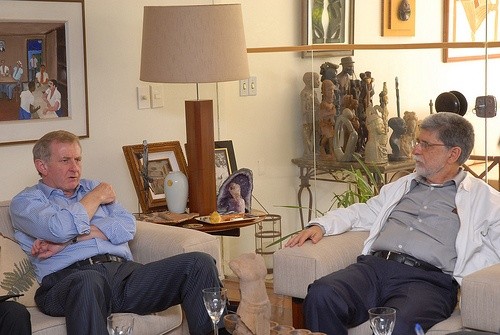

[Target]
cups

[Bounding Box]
[368,307,396,335]
[306,332,327,335]
[270,321,279,335]
[272,325,295,335]
[224,314,238,334]
[107,313,134,335]
[287,329,312,335]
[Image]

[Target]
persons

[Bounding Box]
[302,57,418,166]
[10,130,231,335]
[285,112,500,335]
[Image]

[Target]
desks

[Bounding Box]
[133,209,267,233]
[292,157,416,231]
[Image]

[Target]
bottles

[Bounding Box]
[163,170,188,214]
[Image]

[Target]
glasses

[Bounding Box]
[415,139,447,152]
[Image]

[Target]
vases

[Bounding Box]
[164,170,189,214]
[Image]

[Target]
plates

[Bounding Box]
[216,168,254,212]
[194,214,259,225]
[142,211,199,225]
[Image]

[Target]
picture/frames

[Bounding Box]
[122,141,189,214]
[302,0,355,58]
[443,0,500,62]
[0,0,90,145]
[186,141,238,199]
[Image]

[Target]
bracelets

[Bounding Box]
[72,237,77,243]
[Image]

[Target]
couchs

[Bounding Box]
[0,201,222,335]
[273,230,500,335]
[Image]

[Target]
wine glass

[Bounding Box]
[202,287,228,335]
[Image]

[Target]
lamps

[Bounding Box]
[140,3,250,217]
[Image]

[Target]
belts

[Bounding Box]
[71,254,125,269]
[373,251,442,276]
